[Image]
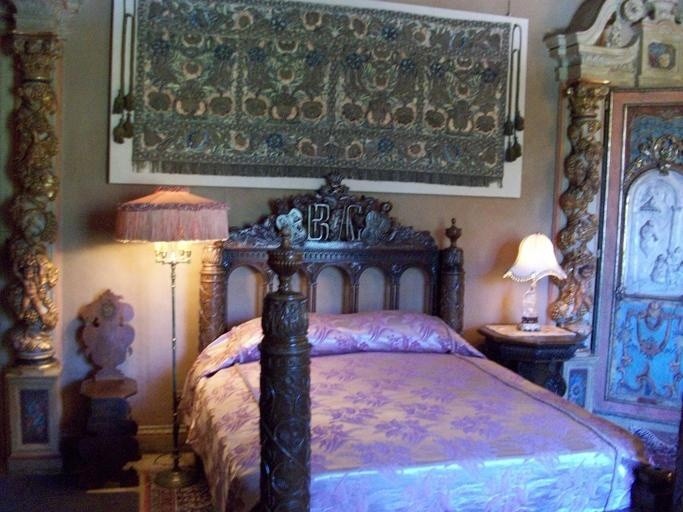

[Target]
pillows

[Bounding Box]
[207,307,479,379]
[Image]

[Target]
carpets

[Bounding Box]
[139,448,216,512]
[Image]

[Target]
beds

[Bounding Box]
[177,173,683,512]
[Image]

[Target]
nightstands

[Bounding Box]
[480,321,584,399]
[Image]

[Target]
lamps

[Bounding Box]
[114,185,228,489]
[505,235,567,333]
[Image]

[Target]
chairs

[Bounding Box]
[79,289,141,488]
